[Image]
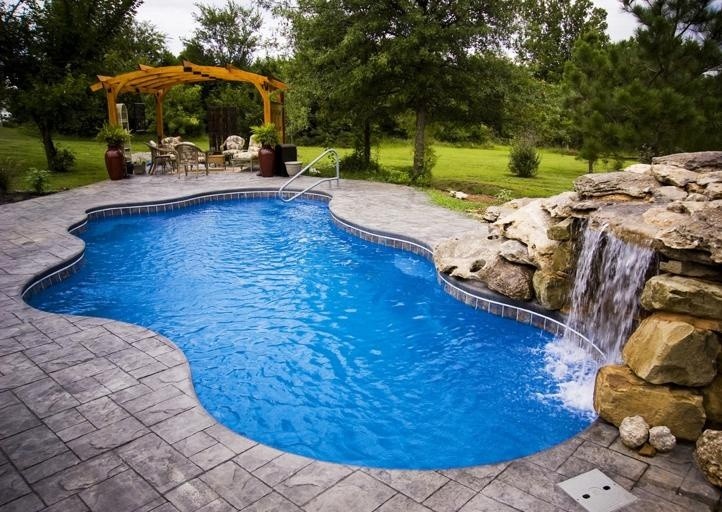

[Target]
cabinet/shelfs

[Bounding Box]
[114,102,133,176]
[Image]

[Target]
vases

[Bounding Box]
[283,160,302,178]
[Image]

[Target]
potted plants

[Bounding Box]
[250,123,279,177]
[95,122,145,180]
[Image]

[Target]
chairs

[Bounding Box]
[143,135,261,180]
